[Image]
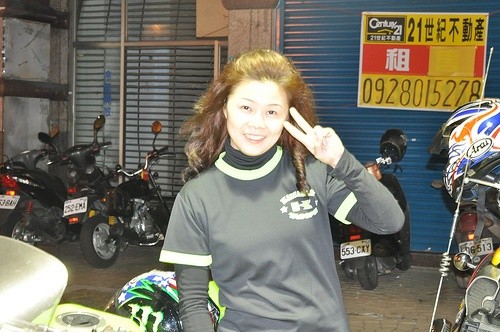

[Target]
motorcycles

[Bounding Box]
[1,234,227,332]
[431,179,500,289]
[62,121,171,269]
[430,98,500,331]
[339,128,412,290]
[0,114,113,246]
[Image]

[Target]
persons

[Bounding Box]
[158,47,406,332]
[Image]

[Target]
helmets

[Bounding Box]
[103,268,220,332]
[429,98,500,201]
[381,128,407,163]
